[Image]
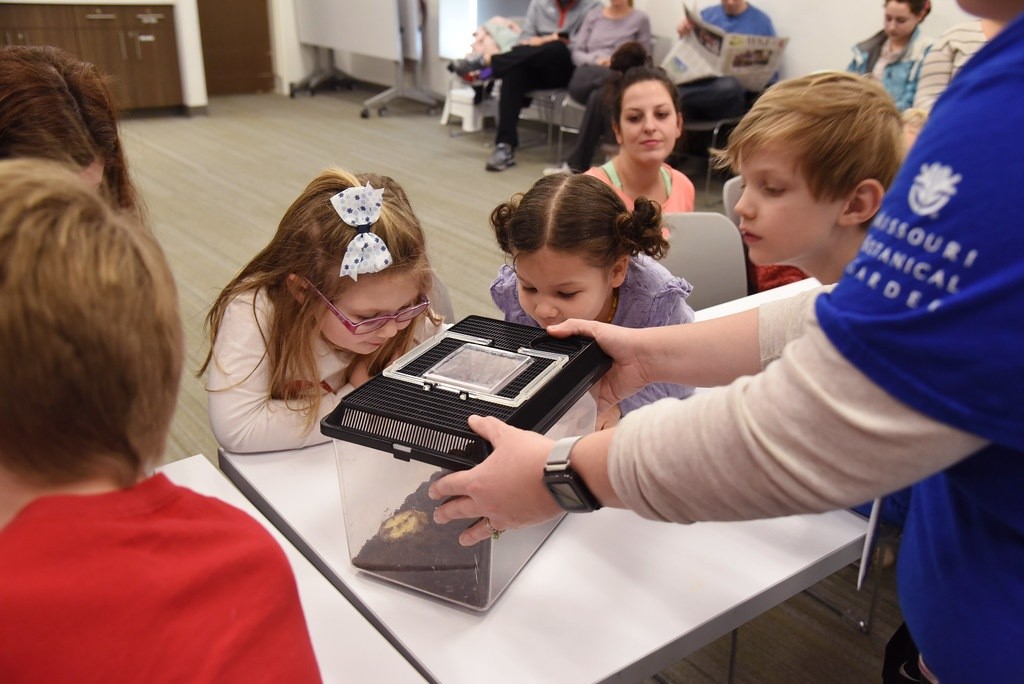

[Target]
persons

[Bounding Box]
[0,44,147,230]
[204,169,453,454]
[0,160,324,684]
[427,0,1024,684]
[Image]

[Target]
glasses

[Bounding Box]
[303,276,430,335]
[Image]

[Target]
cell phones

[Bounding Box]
[558,31,569,40]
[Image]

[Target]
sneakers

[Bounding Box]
[450,54,487,76]
[485,143,515,171]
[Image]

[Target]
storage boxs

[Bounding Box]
[317,314,616,610]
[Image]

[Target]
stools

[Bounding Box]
[439,88,499,132]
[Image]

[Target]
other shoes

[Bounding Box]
[543,162,574,179]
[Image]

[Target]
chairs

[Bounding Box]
[494,89,565,159]
[645,212,748,312]
[682,56,791,207]
[722,175,745,224]
[448,79,500,138]
[556,94,586,168]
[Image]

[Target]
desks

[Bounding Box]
[226,275,870,684]
[162,452,431,684]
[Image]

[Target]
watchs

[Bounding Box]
[542,435,604,513]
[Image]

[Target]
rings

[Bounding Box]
[485,517,506,538]
[607,291,618,323]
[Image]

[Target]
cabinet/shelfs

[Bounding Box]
[0,4,181,114]
